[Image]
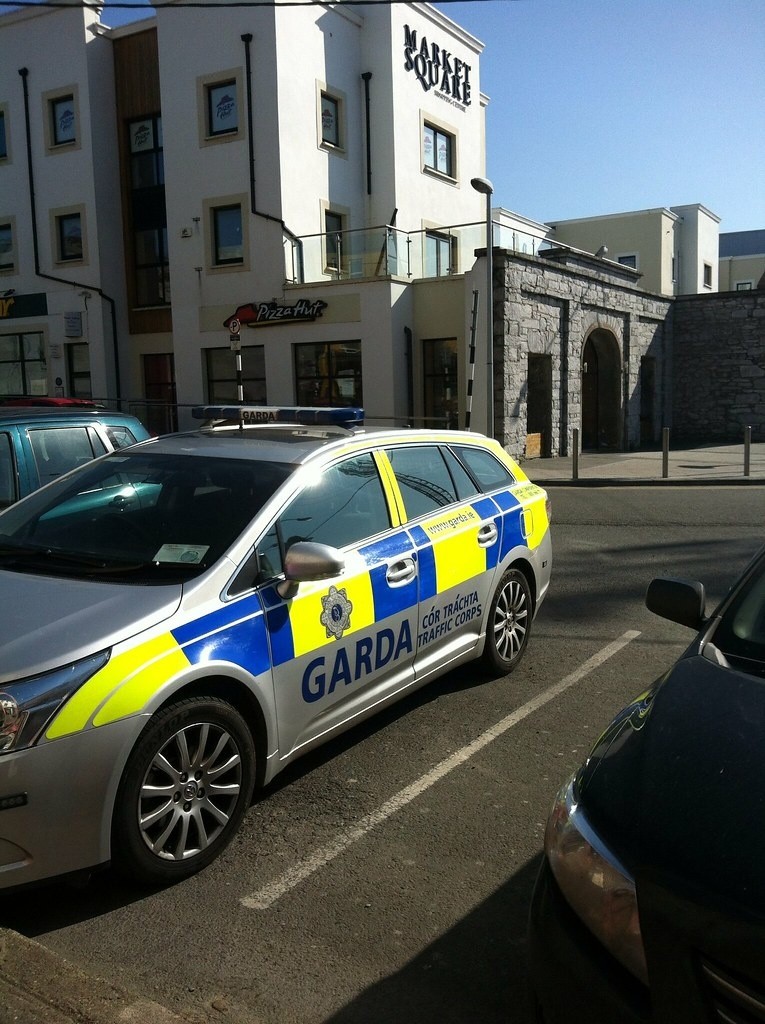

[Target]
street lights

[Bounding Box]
[470,177,497,441]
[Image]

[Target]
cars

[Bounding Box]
[0,395,165,525]
[0,399,552,890]
[525,538,764,1024]
